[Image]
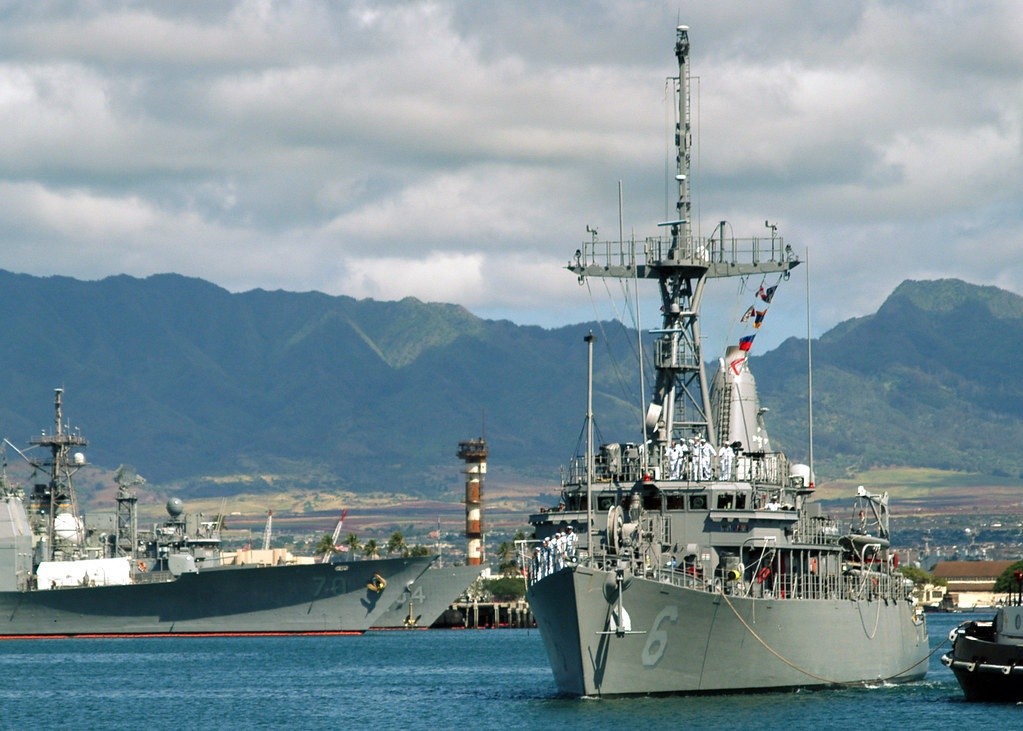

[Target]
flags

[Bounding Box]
[731,285,778,376]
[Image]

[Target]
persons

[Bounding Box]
[664,436,736,481]
[51,580,56,590]
[664,556,677,581]
[541,503,567,514]
[688,562,699,587]
[533,526,578,583]
[758,496,783,511]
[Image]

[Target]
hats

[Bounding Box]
[528,532,566,558]
[568,525,573,529]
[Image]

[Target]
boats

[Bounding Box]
[0,387,496,637]
[941,568,1023,702]
[513,10,932,700]
